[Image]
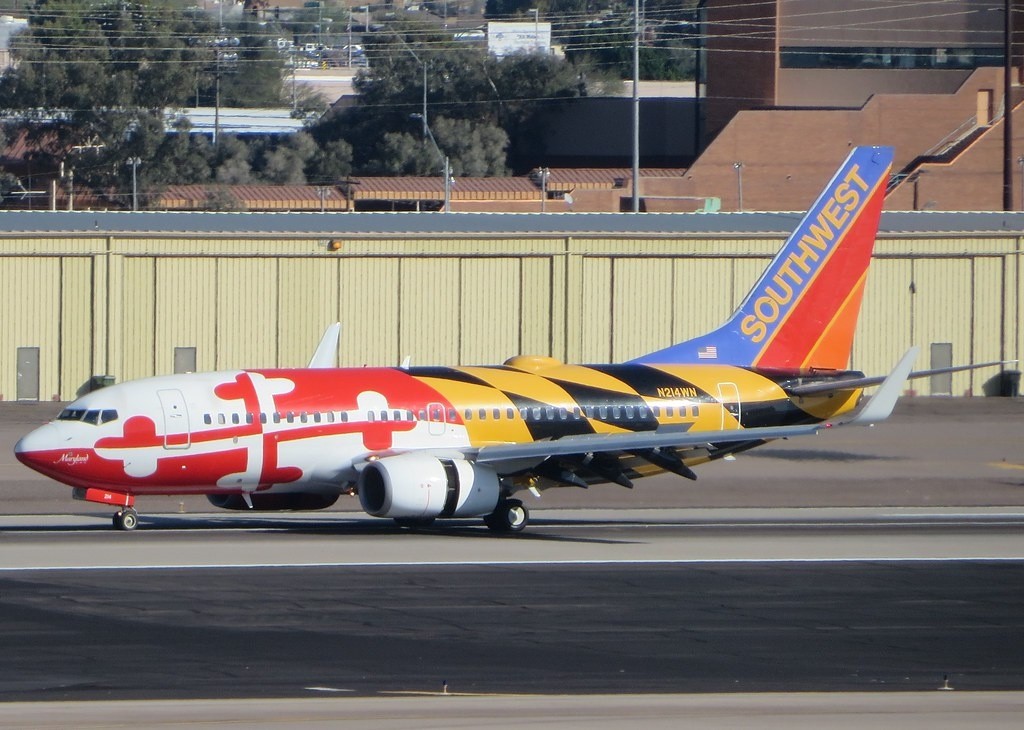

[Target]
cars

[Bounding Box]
[200,31,371,69]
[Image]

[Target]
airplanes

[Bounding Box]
[10,137,1024,539]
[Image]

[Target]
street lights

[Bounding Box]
[359,5,371,32]
[528,8,541,47]
[409,111,452,212]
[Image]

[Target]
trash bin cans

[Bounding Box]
[1000,370,1022,397]
[91,375,115,392]
